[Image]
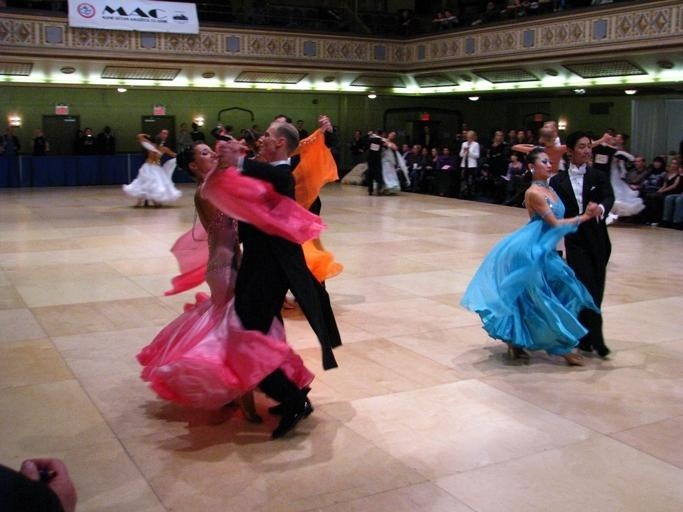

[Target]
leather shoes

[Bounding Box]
[580,342,596,352]
[376,193,381,195]
[595,339,610,358]
[144,203,147,206]
[269,401,313,416]
[368,192,372,194]
[273,398,313,440]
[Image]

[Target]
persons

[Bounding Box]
[295,119,308,138]
[235,122,343,441]
[347,130,366,168]
[650,157,681,226]
[452,132,462,156]
[394,143,411,191]
[404,144,421,192]
[516,128,526,164]
[272,114,323,303]
[121,131,183,208]
[526,128,539,163]
[549,129,616,358]
[418,147,439,193]
[77,127,101,155]
[0,126,21,157]
[526,121,566,186]
[657,160,683,231]
[214,125,250,171]
[511,124,613,186]
[190,122,205,147]
[436,147,453,196]
[410,148,432,193]
[505,167,533,208]
[507,129,516,159]
[588,130,629,222]
[630,156,667,226]
[367,128,388,196]
[240,126,297,311]
[605,131,646,227]
[458,130,480,201]
[29,128,50,155]
[486,130,507,204]
[96,125,117,154]
[209,126,231,141]
[371,130,411,196]
[0,457,77,512]
[492,152,523,205]
[460,145,599,366]
[624,154,649,224]
[135,141,327,426]
[456,129,467,153]
[143,128,169,208]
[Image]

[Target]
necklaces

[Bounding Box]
[530,180,549,188]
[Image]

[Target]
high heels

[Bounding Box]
[225,400,239,407]
[236,387,262,424]
[134,203,142,206]
[561,351,586,367]
[283,300,296,310]
[154,203,159,205]
[508,343,532,361]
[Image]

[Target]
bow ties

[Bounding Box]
[570,168,587,176]
